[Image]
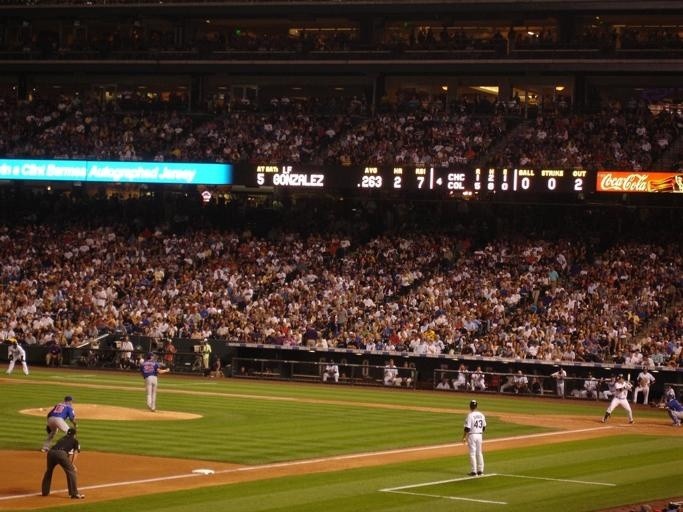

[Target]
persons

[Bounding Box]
[138,352,170,411]
[42,429,85,499]
[322,360,339,383]
[0,190,682,368]
[657,385,676,409]
[436,364,488,391]
[383,359,412,388]
[487,368,567,399]
[664,393,683,426]
[583,372,599,400]
[1,27,683,170]
[602,374,634,423]
[5,341,28,375]
[463,399,487,476]
[633,366,655,405]
[41,397,76,455]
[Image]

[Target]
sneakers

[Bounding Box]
[71,495,85,499]
[468,472,483,476]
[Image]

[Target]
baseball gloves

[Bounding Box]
[9,354,14,360]
[47,425,51,434]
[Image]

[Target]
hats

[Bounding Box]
[64,396,72,402]
[67,429,76,435]
[470,400,477,407]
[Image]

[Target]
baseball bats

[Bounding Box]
[627,374,630,386]
